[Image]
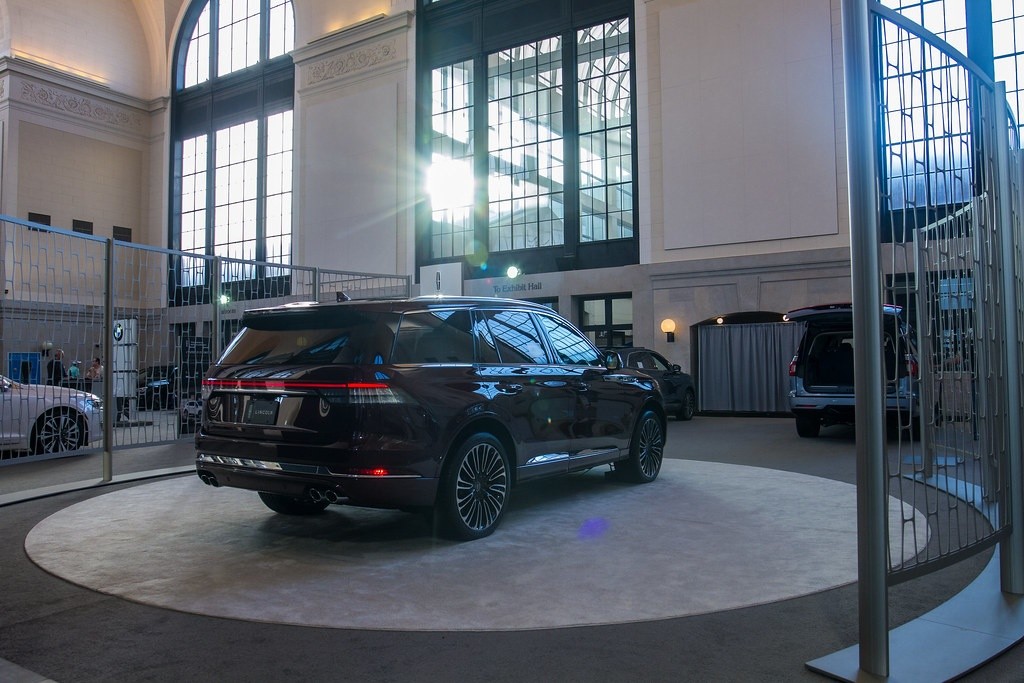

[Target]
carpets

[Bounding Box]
[23,457,931,632]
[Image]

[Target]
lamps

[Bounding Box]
[507,266,521,278]
[661,319,676,343]
[41,341,53,357]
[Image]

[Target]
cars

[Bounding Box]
[599,348,696,421]
[183,401,202,416]
[0,374,103,455]
[122,365,178,421]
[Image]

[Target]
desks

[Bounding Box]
[62,378,91,393]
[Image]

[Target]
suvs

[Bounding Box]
[786,302,939,441]
[193,291,669,542]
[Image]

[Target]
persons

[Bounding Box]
[942,339,979,442]
[47,349,104,386]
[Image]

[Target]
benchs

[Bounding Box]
[817,342,894,383]
